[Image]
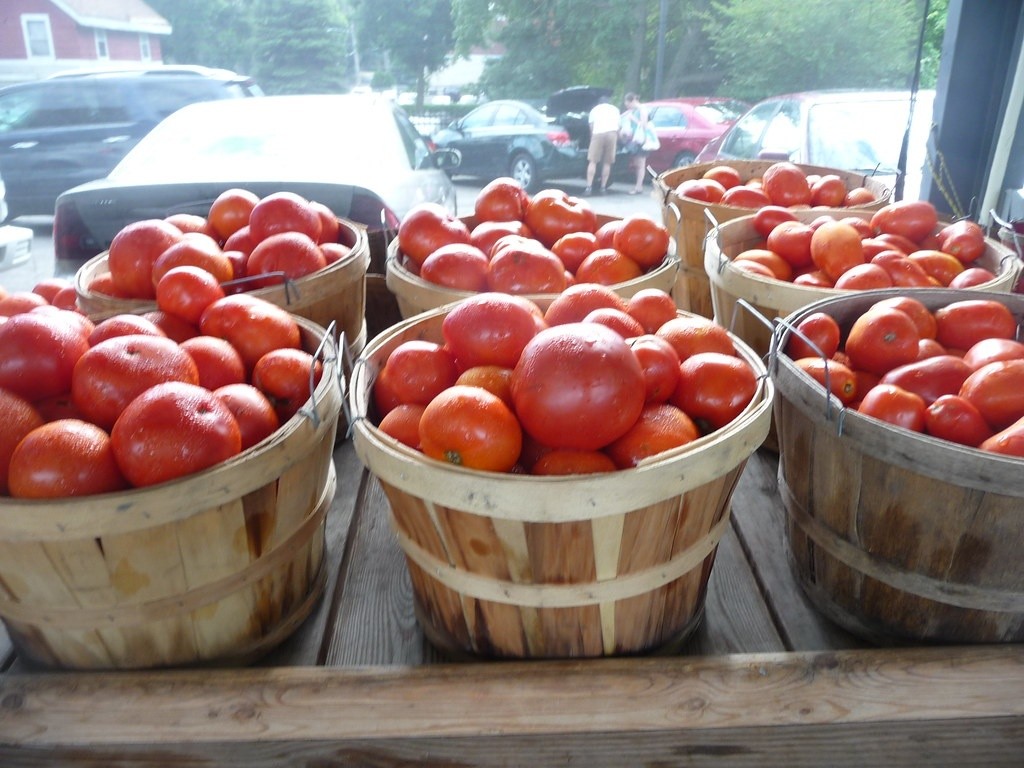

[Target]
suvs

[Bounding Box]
[0,65,268,224]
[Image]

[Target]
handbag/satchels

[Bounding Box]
[626,121,645,154]
[618,116,633,144]
[642,121,661,152]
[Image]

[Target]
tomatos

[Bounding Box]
[0,162,1023,504]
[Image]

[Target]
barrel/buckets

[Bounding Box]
[333,286,771,656]
[376,213,685,316]
[653,156,1024,649]
[0,297,342,669]
[75,224,370,446]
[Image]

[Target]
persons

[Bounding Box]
[624,91,650,195]
[584,95,622,196]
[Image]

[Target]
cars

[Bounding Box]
[612,95,756,183]
[56,91,466,338]
[688,87,1024,294]
[429,84,639,193]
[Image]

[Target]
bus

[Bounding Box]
[0,181,35,284]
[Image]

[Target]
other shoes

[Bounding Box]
[582,186,592,197]
[597,187,607,195]
[630,189,642,194]
